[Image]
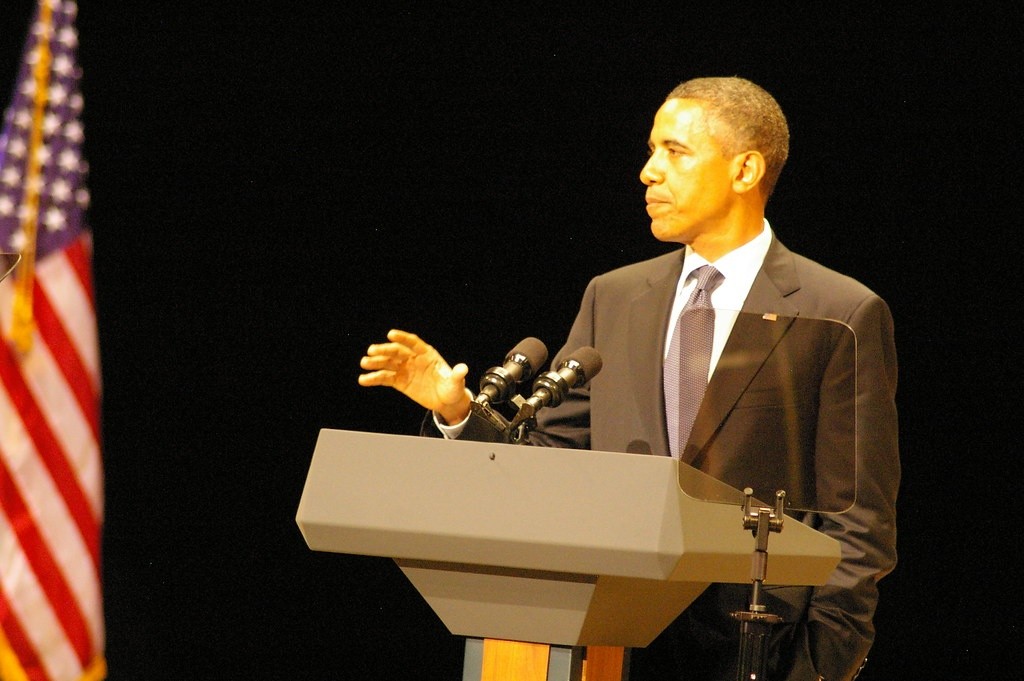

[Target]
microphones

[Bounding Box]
[469,337,548,402]
[534,345,604,407]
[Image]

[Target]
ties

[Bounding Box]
[660,267,723,460]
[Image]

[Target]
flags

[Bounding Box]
[0,0,104,681]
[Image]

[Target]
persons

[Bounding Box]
[360,76,902,681]
